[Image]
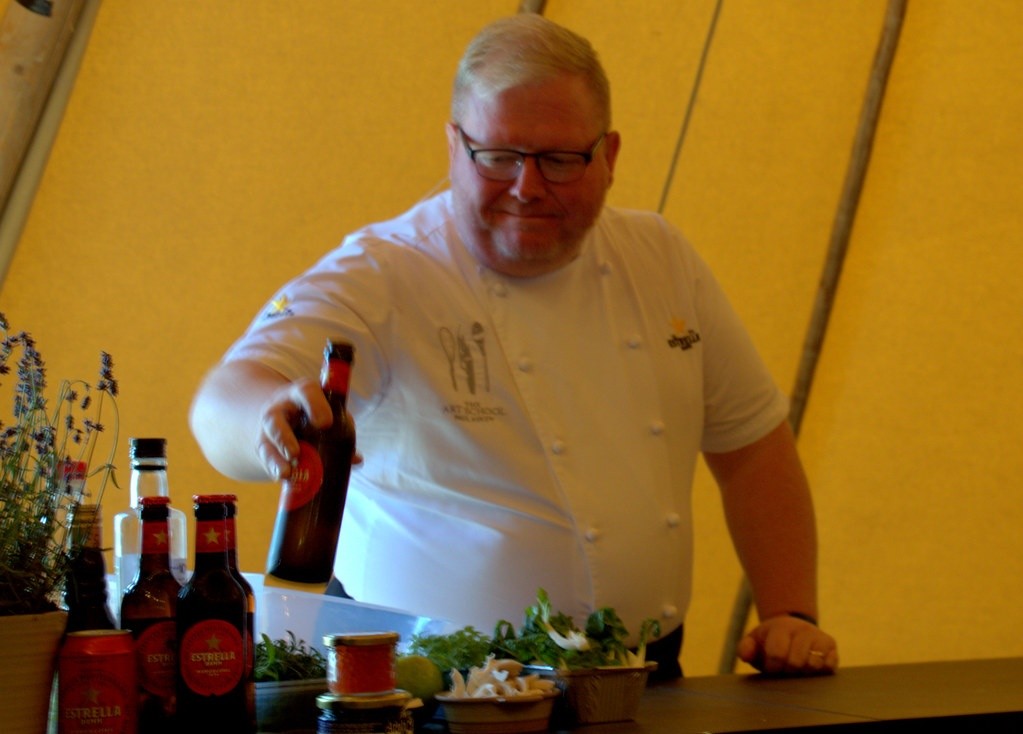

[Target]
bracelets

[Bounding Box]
[788,611,816,625]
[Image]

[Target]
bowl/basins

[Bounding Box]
[520,660,658,725]
[434,687,560,734]
[254,677,328,734]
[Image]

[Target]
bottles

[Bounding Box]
[48,545,118,734]
[45,460,87,547]
[262,336,356,594]
[173,492,263,734]
[117,496,183,733]
[112,437,188,625]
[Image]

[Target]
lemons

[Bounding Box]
[392,654,443,731]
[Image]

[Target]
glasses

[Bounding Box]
[457,127,608,185]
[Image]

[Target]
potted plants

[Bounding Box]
[0,311,122,734]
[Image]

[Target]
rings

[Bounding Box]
[810,651,825,656]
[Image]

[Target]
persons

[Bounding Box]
[189,14,838,678]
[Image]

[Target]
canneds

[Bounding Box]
[316,688,414,734]
[57,629,145,734]
[322,629,400,696]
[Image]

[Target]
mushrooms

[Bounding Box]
[447,651,555,699]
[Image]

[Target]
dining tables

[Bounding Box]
[260,654,1023,734]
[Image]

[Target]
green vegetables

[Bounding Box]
[392,587,661,679]
[253,629,331,734]
